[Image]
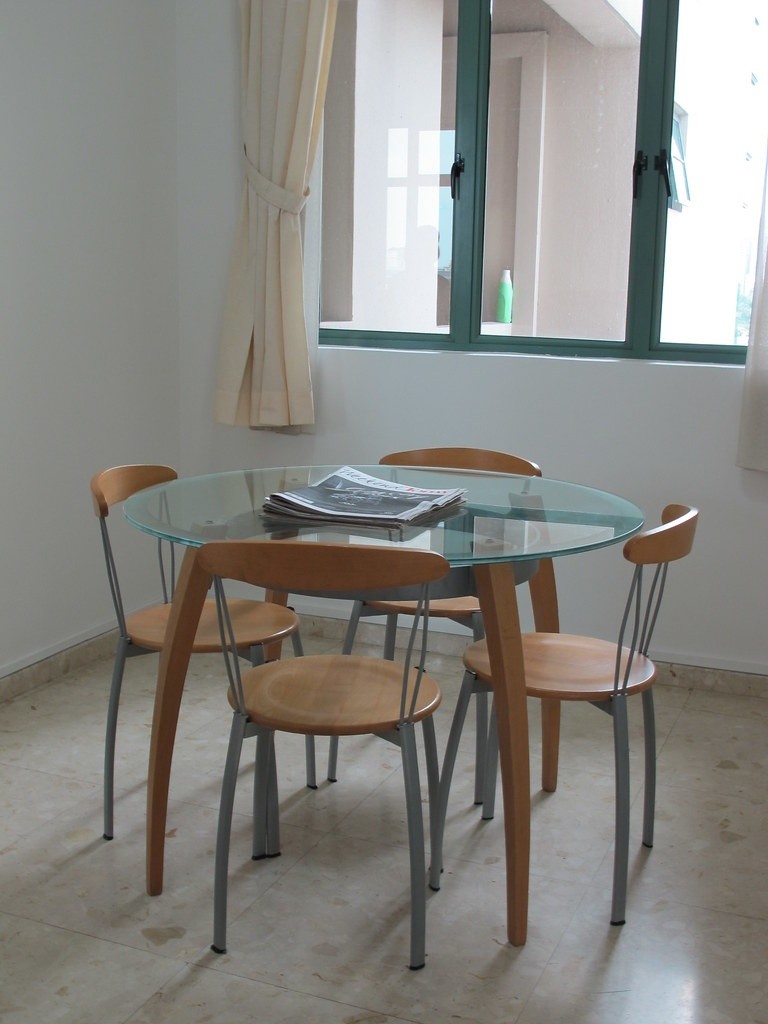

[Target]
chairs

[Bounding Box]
[90,448,700,973]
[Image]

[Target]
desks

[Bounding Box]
[123,466,647,949]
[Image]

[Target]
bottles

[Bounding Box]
[496,267,513,323]
